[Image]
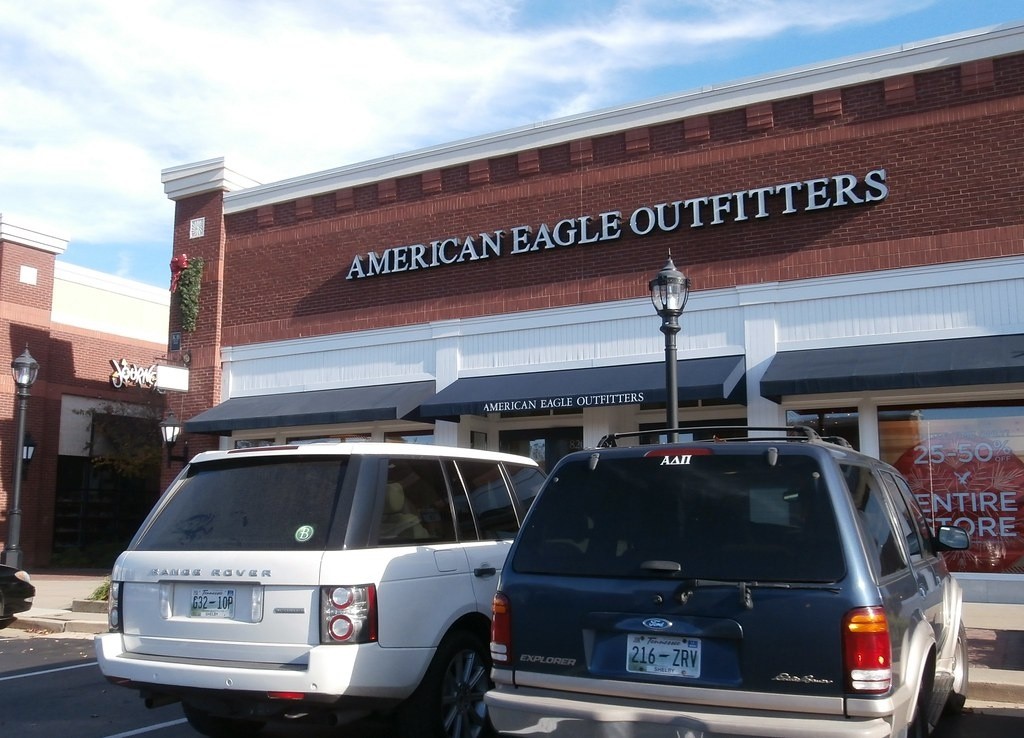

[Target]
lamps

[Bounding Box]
[23,431,36,479]
[159,413,190,467]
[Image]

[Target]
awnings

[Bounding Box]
[759,334,1024,406]
[185,380,436,437]
[419,356,745,423]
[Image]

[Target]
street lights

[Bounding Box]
[645,248,691,440]
[4,342,41,566]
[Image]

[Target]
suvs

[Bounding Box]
[483,423,972,738]
[92,440,631,738]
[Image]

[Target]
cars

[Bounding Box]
[0,560,36,633]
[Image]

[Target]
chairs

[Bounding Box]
[379,482,433,543]
